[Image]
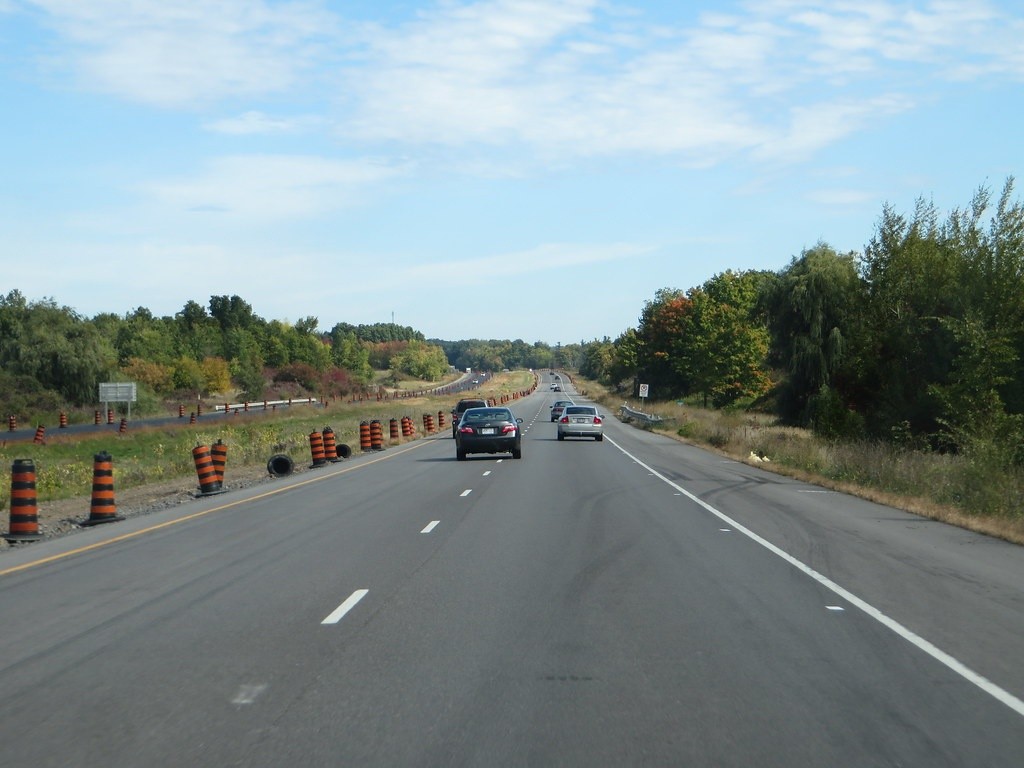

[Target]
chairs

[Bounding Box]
[470,414,479,419]
[495,414,505,419]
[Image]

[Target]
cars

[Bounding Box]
[473,379,478,384]
[556,405,606,442]
[550,401,576,422]
[554,387,561,392]
[451,398,489,439]
[452,408,523,461]
[550,383,558,389]
